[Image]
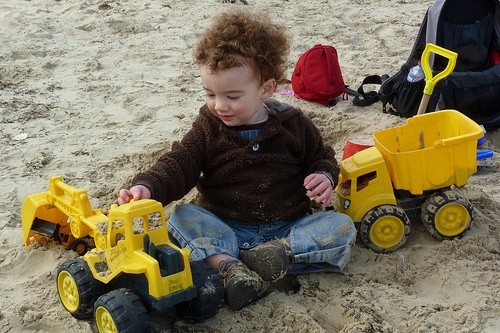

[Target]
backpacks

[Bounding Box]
[378,0,500,132]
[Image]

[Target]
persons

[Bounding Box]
[115,5,360,309]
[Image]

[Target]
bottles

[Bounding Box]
[406,60,425,82]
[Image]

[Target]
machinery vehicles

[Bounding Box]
[339,109,486,255]
[22,174,226,332]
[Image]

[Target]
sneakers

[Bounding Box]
[217,261,272,312]
[239,242,290,284]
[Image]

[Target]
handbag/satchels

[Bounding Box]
[291,43,345,110]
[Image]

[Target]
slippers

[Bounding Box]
[353,72,391,108]
